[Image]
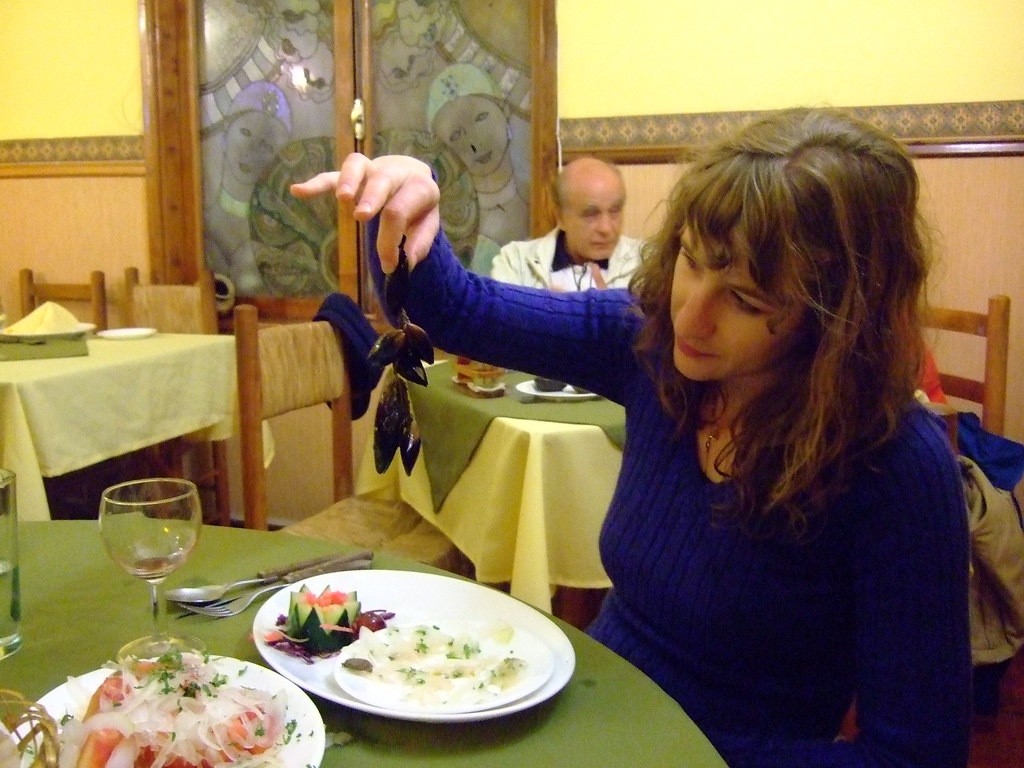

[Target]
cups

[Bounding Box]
[0,467,22,661]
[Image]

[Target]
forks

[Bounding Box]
[177,559,372,617]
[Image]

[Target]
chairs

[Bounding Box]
[19,269,118,520]
[233,304,473,577]
[125,267,231,527]
[918,295,1010,441]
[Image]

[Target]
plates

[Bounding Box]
[11,655,326,768]
[516,375,602,403]
[1,322,97,343]
[252,570,576,723]
[333,624,552,713]
[96,327,158,341]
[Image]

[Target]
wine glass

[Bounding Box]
[96,478,207,663]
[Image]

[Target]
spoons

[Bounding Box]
[162,549,345,603]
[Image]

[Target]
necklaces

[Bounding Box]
[703,401,716,474]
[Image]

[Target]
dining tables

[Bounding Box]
[0,334,239,522]
[355,359,628,615]
[0,520,729,768]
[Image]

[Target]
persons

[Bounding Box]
[491,156,661,293]
[290,107,971,768]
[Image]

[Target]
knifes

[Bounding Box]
[174,549,375,618]
